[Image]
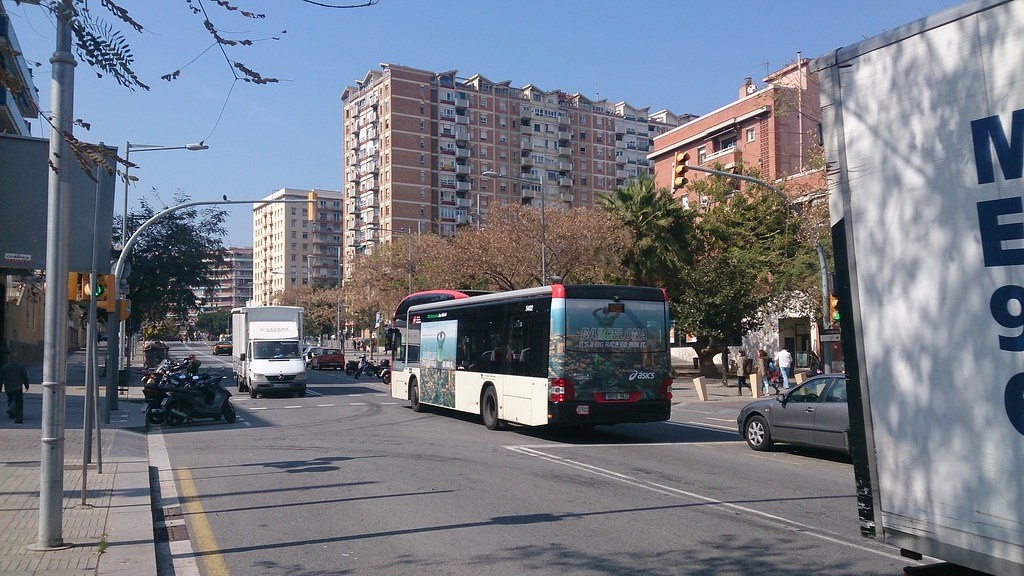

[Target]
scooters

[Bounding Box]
[140,354,237,423]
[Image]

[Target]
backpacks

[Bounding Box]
[769,362,775,372]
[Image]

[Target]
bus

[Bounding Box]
[384,283,675,440]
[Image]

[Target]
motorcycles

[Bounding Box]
[345,352,391,384]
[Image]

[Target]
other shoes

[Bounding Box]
[775,389,780,395]
[736,393,743,396]
[766,393,770,396]
[6,409,14,419]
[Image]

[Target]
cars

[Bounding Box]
[302,345,345,371]
[737,373,851,455]
[211,341,233,356]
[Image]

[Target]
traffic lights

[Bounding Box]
[673,151,692,190]
[81,272,91,301]
[89,274,116,313]
[829,292,840,326]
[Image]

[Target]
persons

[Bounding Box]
[188,354,201,376]
[0,351,29,418]
[809,351,821,377]
[353,340,364,351]
[753,345,793,396]
[734,349,752,396]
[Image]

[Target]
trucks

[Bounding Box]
[231,305,309,398]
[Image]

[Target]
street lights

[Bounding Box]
[367,225,413,294]
[122,140,210,250]
[109,195,317,411]
[672,162,831,331]
[482,171,546,286]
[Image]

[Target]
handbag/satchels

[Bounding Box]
[817,369,823,374]
